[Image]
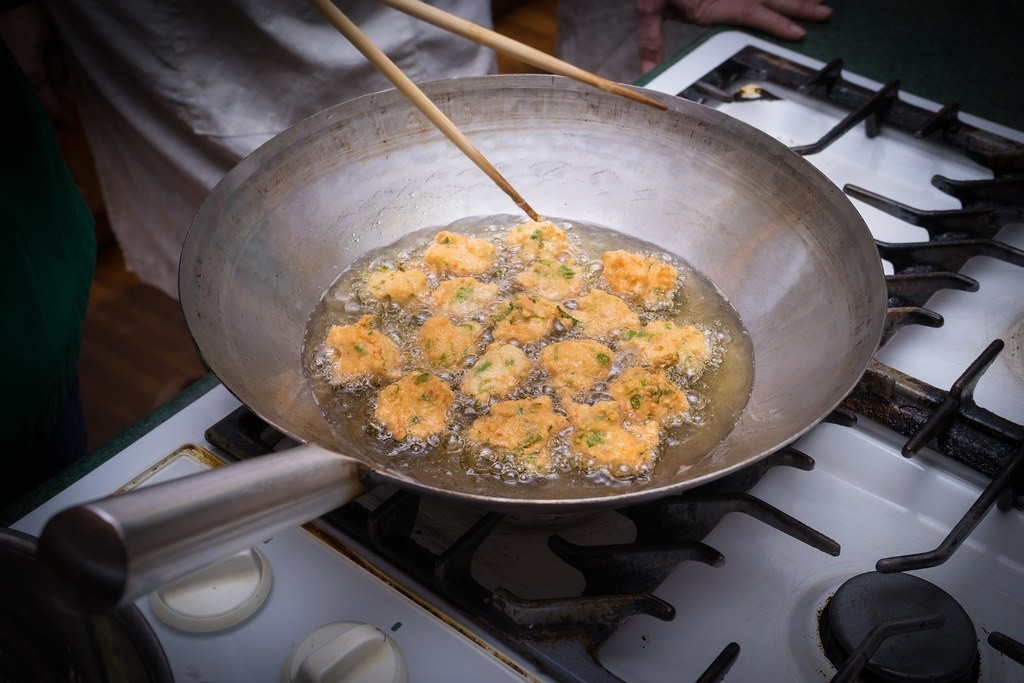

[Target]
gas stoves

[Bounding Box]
[0,27,1022,683]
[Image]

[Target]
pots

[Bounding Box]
[27,73,889,615]
[1,523,180,682]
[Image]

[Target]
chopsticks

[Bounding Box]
[308,1,668,224]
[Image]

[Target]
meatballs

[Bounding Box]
[325,222,707,480]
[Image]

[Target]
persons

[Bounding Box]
[46,2,834,372]
